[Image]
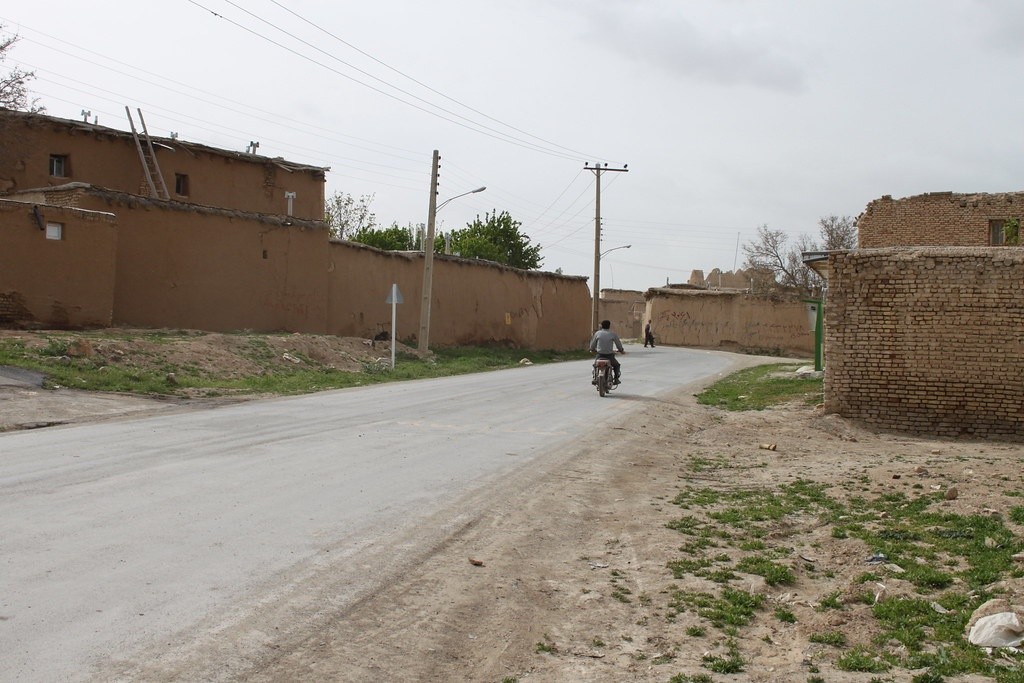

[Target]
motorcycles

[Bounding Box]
[589,348,625,397]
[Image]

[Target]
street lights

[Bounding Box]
[591,244,632,339]
[419,187,487,351]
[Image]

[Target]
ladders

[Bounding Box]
[125,106,170,200]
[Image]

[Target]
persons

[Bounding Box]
[644,320,655,347]
[590,320,624,384]
